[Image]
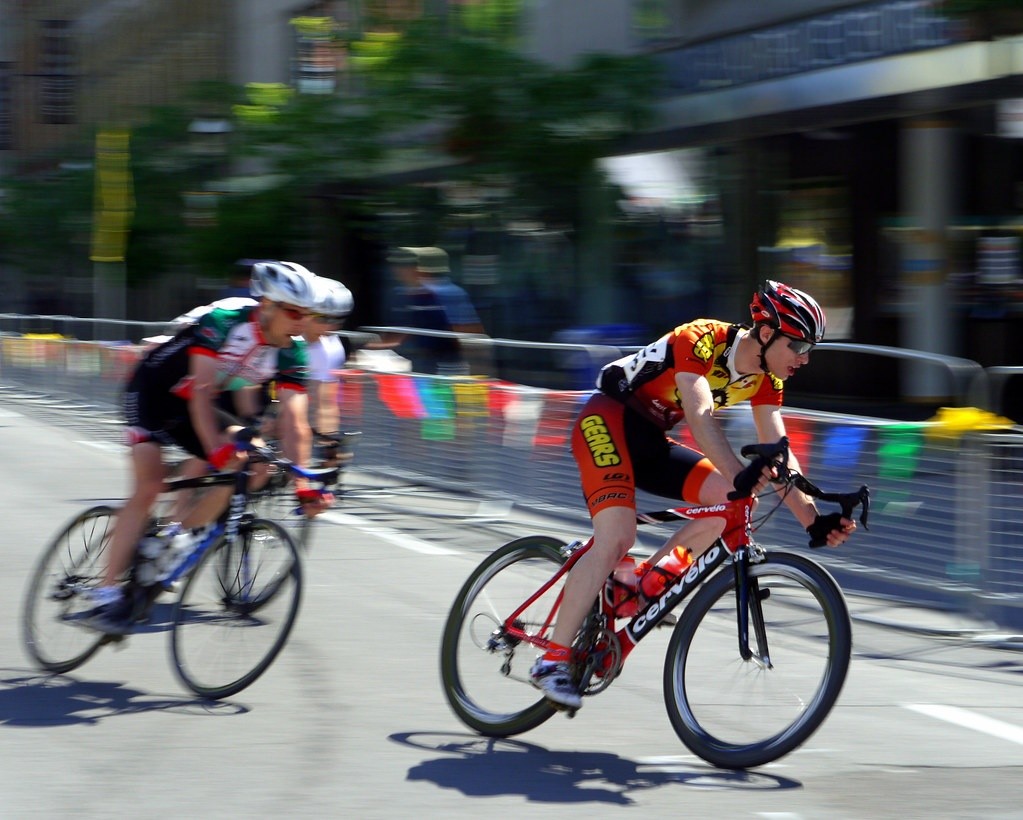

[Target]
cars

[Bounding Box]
[770,342,986,423]
[982,365,1023,426]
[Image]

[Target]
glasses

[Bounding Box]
[780,332,817,356]
[313,313,347,326]
[274,303,310,324]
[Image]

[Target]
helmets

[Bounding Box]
[248,262,316,307]
[750,278,827,345]
[307,272,354,317]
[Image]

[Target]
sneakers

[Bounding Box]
[529,658,583,710]
[639,598,677,629]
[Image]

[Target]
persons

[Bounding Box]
[526,279,856,711]
[363,247,484,379]
[58,258,354,635]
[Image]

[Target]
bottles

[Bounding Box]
[157,528,201,581]
[641,546,693,598]
[136,532,160,587]
[613,554,640,617]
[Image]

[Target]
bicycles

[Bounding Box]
[21,408,342,700]
[438,435,870,767]
[149,410,361,610]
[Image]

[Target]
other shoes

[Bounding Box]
[63,590,126,635]
